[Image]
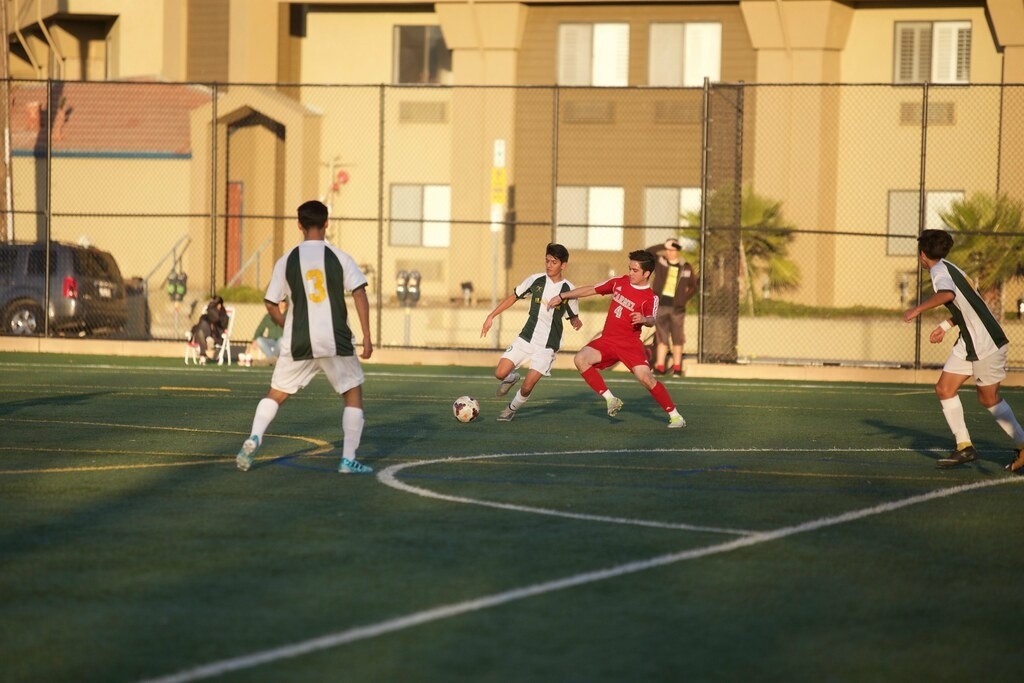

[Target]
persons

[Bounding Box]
[251,294,290,364]
[904,230,1024,473]
[236,200,374,473]
[194,296,228,366]
[480,243,582,421]
[645,238,697,379]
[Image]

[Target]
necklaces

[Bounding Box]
[548,250,687,428]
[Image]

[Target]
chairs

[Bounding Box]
[184,304,235,366]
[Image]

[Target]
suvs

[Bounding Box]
[0,238,128,337]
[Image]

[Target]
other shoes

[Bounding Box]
[651,368,664,377]
[673,370,681,379]
[199,356,207,367]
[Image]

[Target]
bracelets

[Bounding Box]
[559,294,563,300]
[940,320,952,332]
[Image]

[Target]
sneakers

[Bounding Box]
[606,398,624,417]
[497,372,521,396]
[338,459,373,473]
[1004,447,1024,473]
[235,437,260,472]
[667,416,687,429]
[496,404,516,422]
[937,444,977,468]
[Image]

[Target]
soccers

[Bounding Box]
[453,395,480,423]
